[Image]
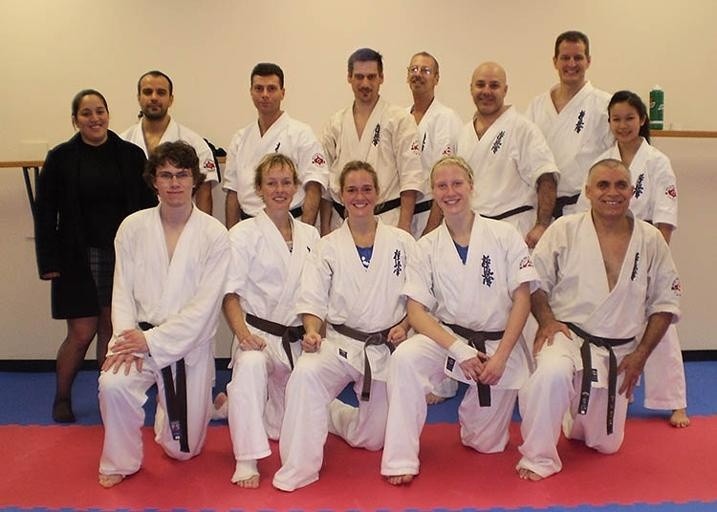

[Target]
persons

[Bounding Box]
[272,160,417,493]
[318,49,423,236]
[119,70,218,216]
[213,153,324,486]
[458,62,560,253]
[36,91,159,421]
[525,30,615,221]
[399,52,460,405]
[381,158,542,488]
[224,65,329,229]
[578,92,691,428]
[97,141,230,489]
[515,159,682,481]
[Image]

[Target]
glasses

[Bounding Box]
[406,67,434,75]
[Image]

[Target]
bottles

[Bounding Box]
[649,85,665,129]
